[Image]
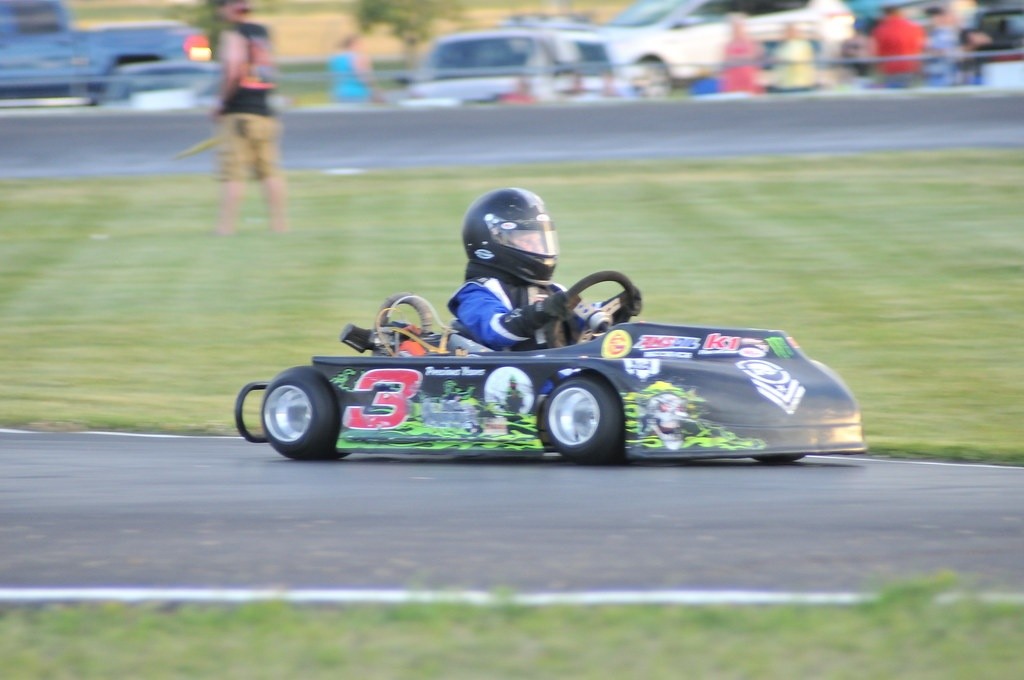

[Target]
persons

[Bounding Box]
[329,34,375,104]
[212,0,288,234]
[446,187,601,350]
[721,5,993,92]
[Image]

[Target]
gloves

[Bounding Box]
[601,286,642,324]
[523,288,575,329]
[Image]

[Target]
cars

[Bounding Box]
[586,0,856,99]
[394,25,634,102]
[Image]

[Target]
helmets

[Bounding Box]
[462,187,558,286]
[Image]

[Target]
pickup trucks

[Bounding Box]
[0,0,214,98]
